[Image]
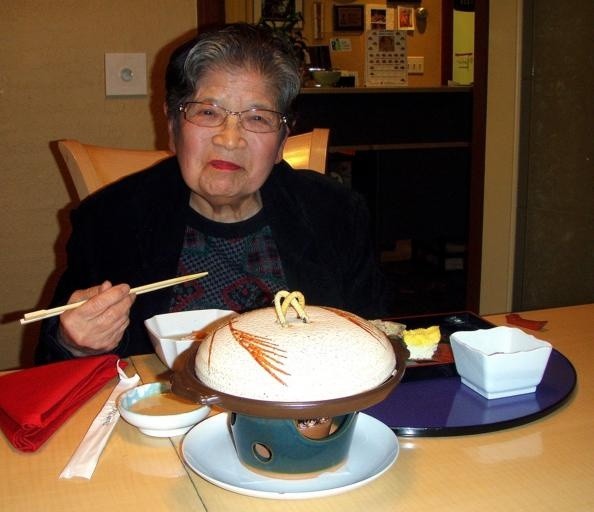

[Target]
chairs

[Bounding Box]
[53,126,331,203]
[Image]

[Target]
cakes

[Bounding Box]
[403,326,441,361]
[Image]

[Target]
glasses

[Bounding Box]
[178,102,289,133]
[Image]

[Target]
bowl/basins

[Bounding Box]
[143,307,240,373]
[117,380,211,439]
[448,326,554,401]
[312,71,342,88]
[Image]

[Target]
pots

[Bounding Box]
[171,304,411,421]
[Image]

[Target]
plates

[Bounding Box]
[180,410,401,501]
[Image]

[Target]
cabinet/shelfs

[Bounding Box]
[284,89,472,322]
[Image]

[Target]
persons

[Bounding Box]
[31,21,378,369]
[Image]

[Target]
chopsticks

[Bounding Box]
[18,271,210,326]
